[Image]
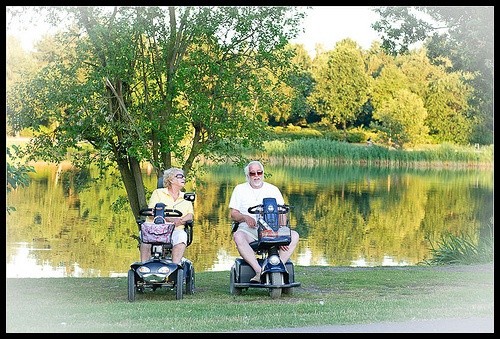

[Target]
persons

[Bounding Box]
[138,167,194,264]
[227,161,299,283]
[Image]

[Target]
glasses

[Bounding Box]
[249,170,263,176]
[173,174,187,179]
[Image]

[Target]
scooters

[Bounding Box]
[228,197,301,299]
[127,192,196,299]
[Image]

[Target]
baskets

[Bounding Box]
[141,216,175,244]
[256,207,290,242]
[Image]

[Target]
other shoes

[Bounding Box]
[250,273,261,284]
